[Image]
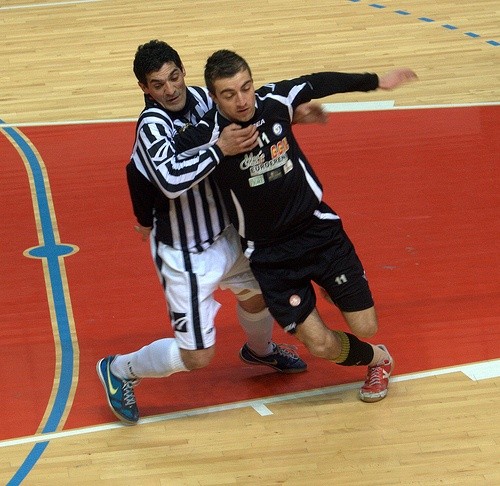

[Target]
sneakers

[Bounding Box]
[96,353,139,424]
[240,341,307,372]
[359,344,396,402]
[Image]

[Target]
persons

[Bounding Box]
[96,39,329,426]
[125,49,418,403]
